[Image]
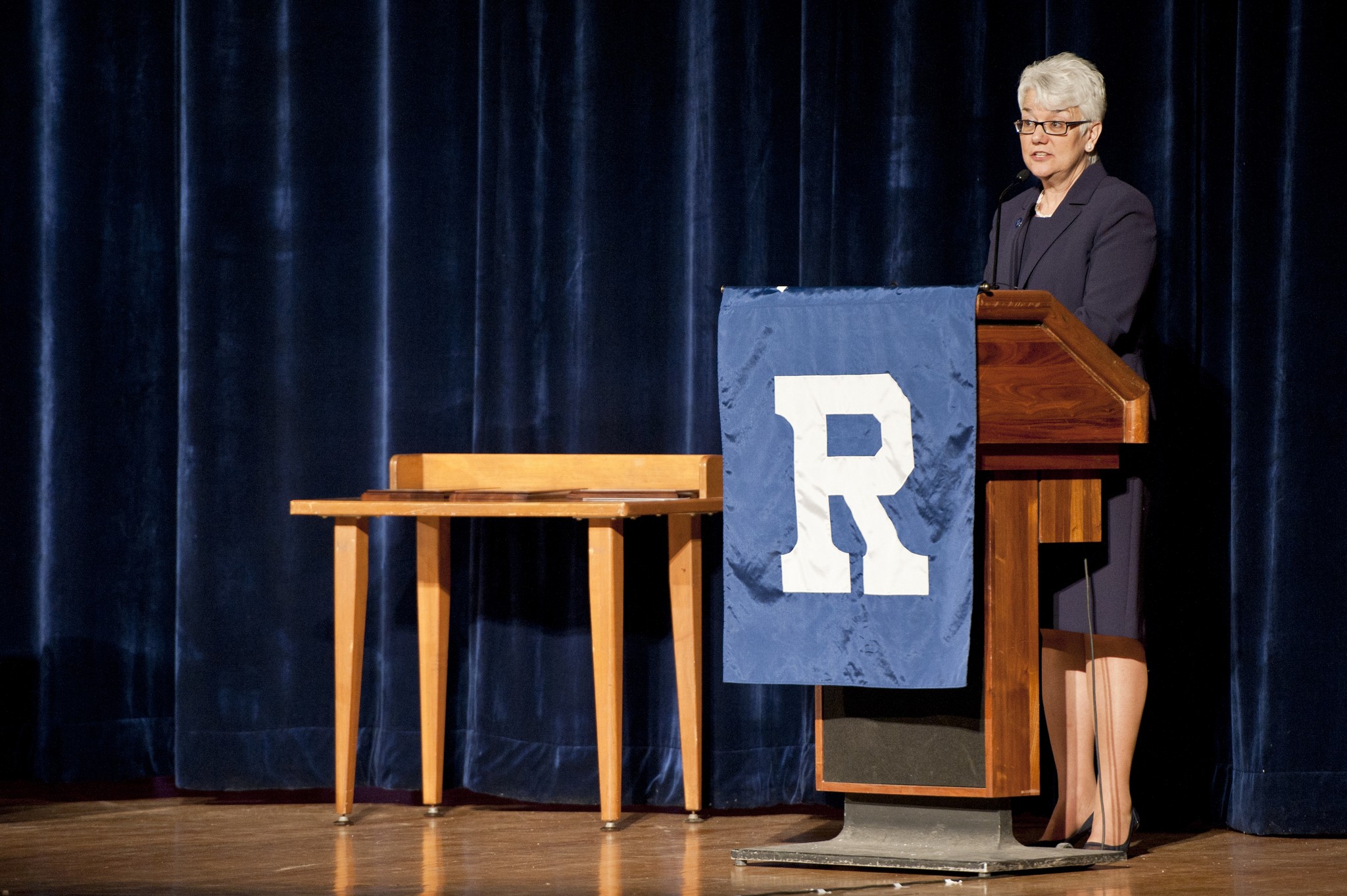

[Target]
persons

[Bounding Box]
[983,53,1156,853]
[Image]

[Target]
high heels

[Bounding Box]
[1084,808,1139,852]
[1032,813,1093,849]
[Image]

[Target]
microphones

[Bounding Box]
[990,167,1030,290]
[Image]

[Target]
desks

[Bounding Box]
[289,453,723,832]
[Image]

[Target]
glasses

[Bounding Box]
[1014,120,1092,135]
